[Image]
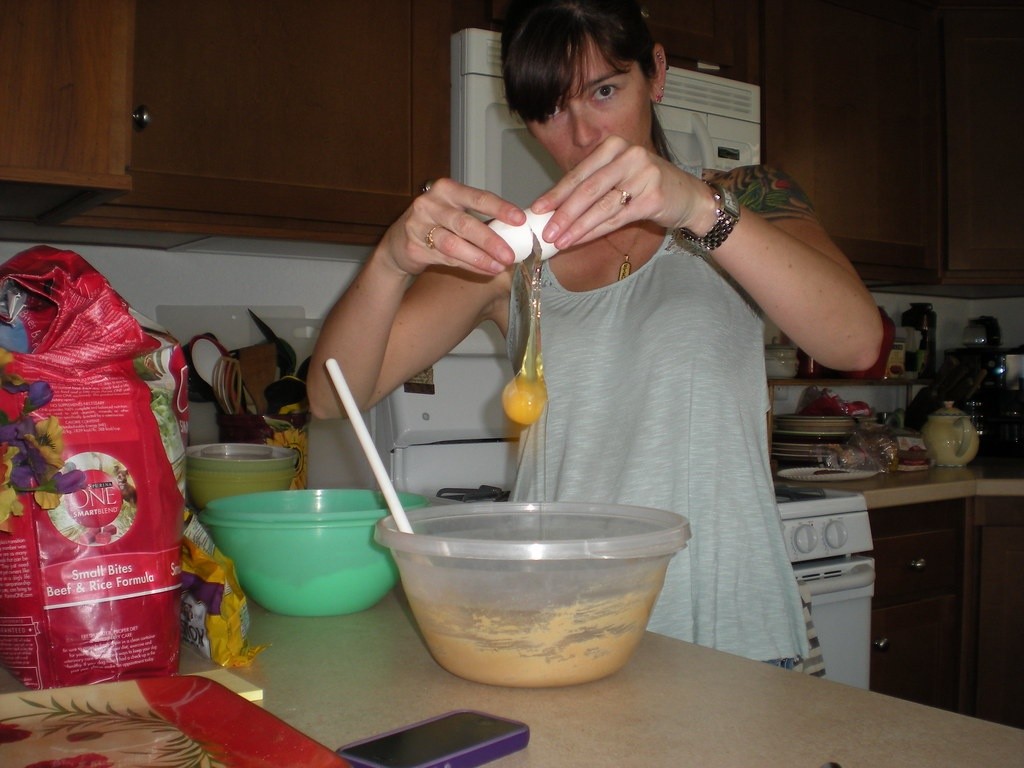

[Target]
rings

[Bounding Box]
[612,186,632,206]
[424,225,443,249]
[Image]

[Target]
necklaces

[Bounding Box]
[605,236,637,283]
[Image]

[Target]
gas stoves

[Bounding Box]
[391,440,874,523]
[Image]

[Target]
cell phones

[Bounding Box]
[335,711,529,768]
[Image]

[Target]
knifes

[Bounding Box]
[927,348,987,409]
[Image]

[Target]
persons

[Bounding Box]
[309,0,885,671]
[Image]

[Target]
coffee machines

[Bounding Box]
[944,347,1024,459]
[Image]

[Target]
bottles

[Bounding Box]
[764,300,939,381]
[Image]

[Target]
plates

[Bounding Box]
[769,412,881,482]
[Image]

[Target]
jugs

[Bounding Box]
[962,314,1002,346]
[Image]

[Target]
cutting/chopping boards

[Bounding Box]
[154,303,306,447]
[249,315,378,489]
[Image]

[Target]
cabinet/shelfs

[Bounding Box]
[854,493,1024,729]
[0,0,1024,295]
[766,379,923,470]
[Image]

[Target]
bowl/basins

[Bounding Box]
[374,501,693,688]
[204,489,433,517]
[201,442,274,461]
[199,510,399,617]
[184,444,300,509]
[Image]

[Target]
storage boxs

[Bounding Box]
[884,326,908,379]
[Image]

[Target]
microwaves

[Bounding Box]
[448,28,762,227]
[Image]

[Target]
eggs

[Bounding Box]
[502,255,548,426]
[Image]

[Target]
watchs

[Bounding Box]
[679,178,741,250]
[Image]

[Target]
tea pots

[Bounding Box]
[922,399,980,468]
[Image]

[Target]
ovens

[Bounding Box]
[781,512,877,691]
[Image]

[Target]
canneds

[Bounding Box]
[797,346,830,378]
